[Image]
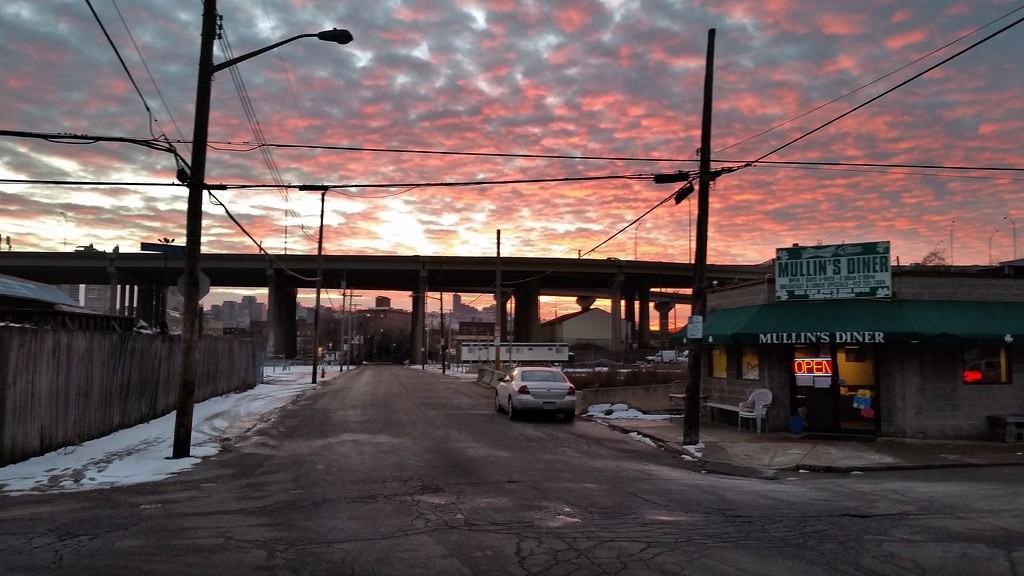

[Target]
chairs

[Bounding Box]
[738,388,772,434]
[669,379,686,423]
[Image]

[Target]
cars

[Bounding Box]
[584,359,611,368]
[494,367,576,423]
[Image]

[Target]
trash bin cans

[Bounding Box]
[789,415,803,434]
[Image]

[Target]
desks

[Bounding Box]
[669,394,708,424]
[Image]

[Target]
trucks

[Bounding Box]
[646,350,676,365]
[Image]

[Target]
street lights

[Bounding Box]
[172,27,356,459]
[408,290,446,374]
[346,312,372,370]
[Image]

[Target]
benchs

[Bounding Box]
[703,403,739,427]
[985,415,1024,443]
